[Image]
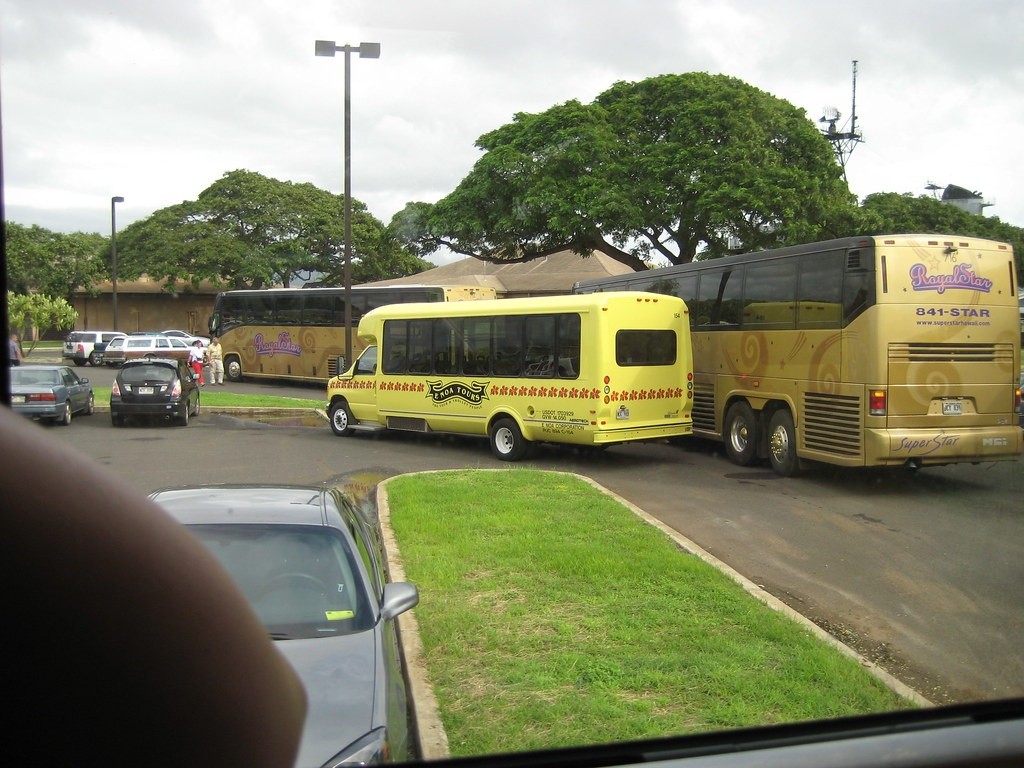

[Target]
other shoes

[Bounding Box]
[210,383,216,386]
[218,382,224,386]
[200,383,205,387]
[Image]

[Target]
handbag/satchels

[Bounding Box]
[195,356,202,361]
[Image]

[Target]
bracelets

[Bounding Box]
[187,361,191,363]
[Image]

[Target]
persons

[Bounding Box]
[9,333,25,367]
[206,337,224,386]
[186,340,207,386]
[0,406,309,768]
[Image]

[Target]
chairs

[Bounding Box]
[433,352,499,375]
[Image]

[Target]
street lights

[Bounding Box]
[315,40,381,376]
[111,196,125,332]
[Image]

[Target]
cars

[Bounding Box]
[142,481,420,767]
[124,330,159,346]
[11,363,95,425]
[108,357,201,426]
[102,333,206,369]
[155,328,211,350]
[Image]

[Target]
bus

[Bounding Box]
[206,283,499,385]
[323,289,696,462]
[575,233,1024,475]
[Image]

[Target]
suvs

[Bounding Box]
[61,331,130,366]
